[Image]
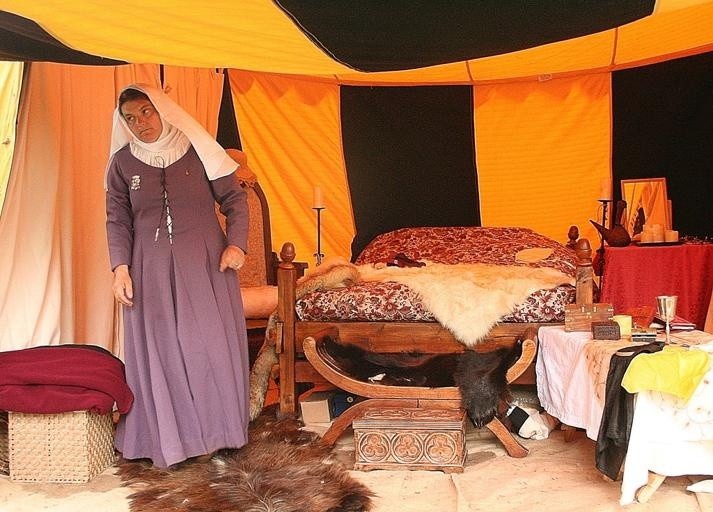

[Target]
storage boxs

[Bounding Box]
[565,303,614,333]
[352,406,468,474]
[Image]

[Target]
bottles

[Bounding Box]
[640,223,664,242]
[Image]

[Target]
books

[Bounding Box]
[654,312,697,330]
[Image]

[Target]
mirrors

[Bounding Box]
[622,178,670,243]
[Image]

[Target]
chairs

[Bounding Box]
[215,180,308,408]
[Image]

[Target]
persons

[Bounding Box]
[101,81,252,471]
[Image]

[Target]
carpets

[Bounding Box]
[112,404,378,511]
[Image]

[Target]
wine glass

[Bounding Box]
[656,296,678,345]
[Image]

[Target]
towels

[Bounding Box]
[620,345,712,406]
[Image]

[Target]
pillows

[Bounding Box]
[354,226,579,278]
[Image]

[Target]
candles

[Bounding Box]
[638,223,679,242]
[600,177,611,201]
[313,180,323,208]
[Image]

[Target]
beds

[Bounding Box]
[277,226,594,417]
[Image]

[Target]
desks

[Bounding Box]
[595,239,713,337]
[537,309,713,504]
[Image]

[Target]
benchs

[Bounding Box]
[302,326,539,474]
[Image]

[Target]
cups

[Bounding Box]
[665,230,679,243]
[612,315,632,338]
